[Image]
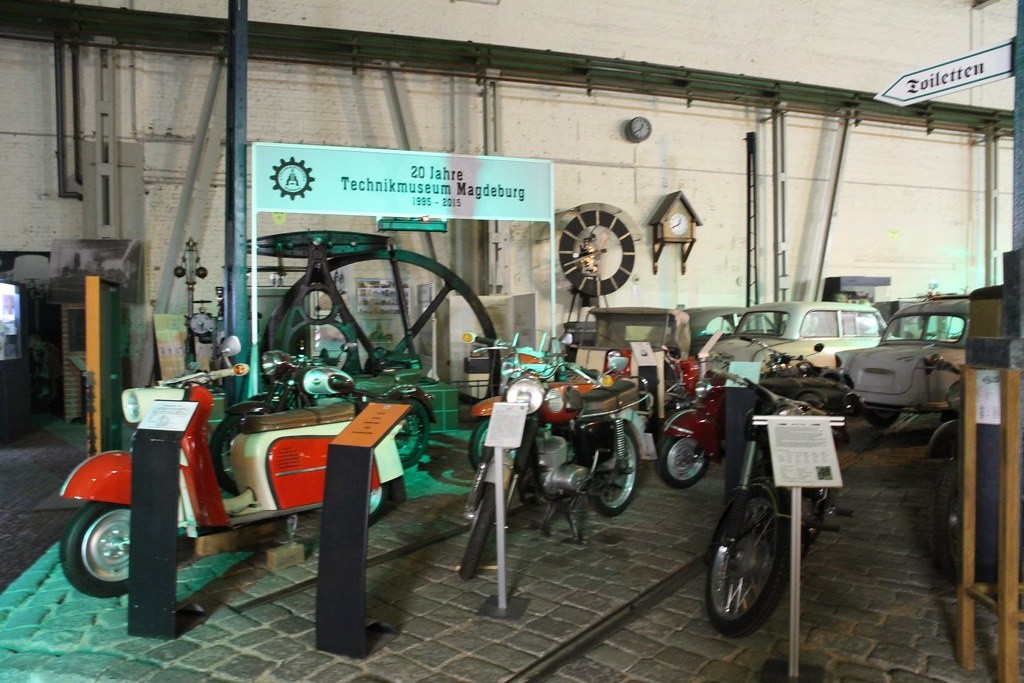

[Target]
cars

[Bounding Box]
[699,301,903,374]
[833,298,970,428]
[647,306,777,358]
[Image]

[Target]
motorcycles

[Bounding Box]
[572,305,861,489]
[704,367,861,638]
[459,328,628,472]
[57,331,436,599]
[926,353,962,583]
[456,337,651,580]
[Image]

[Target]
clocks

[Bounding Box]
[626,116,652,143]
[670,213,689,235]
